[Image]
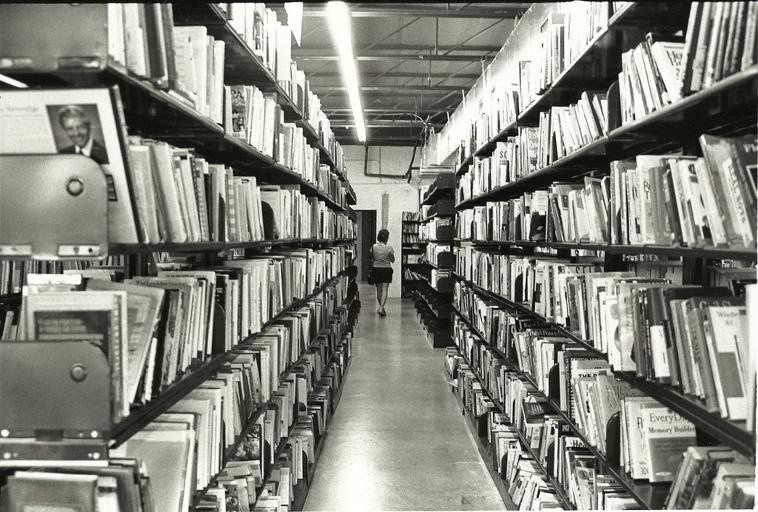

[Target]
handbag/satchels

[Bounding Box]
[367,268,374,286]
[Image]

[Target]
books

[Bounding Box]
[1,2,363,512]
[404,1,756,511]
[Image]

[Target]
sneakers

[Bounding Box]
[377,307,387,317]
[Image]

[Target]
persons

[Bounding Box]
[369,228,395,317]
